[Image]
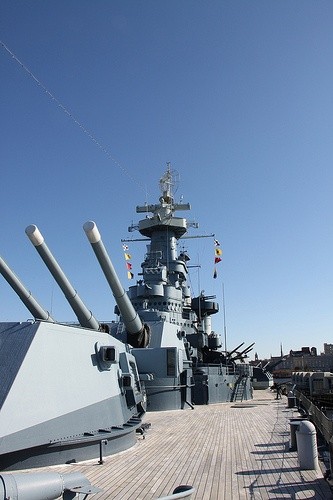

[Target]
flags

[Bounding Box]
[122,243,134,280]
[212,239,223,279]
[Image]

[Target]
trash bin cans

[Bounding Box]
[295,421,319,471]
